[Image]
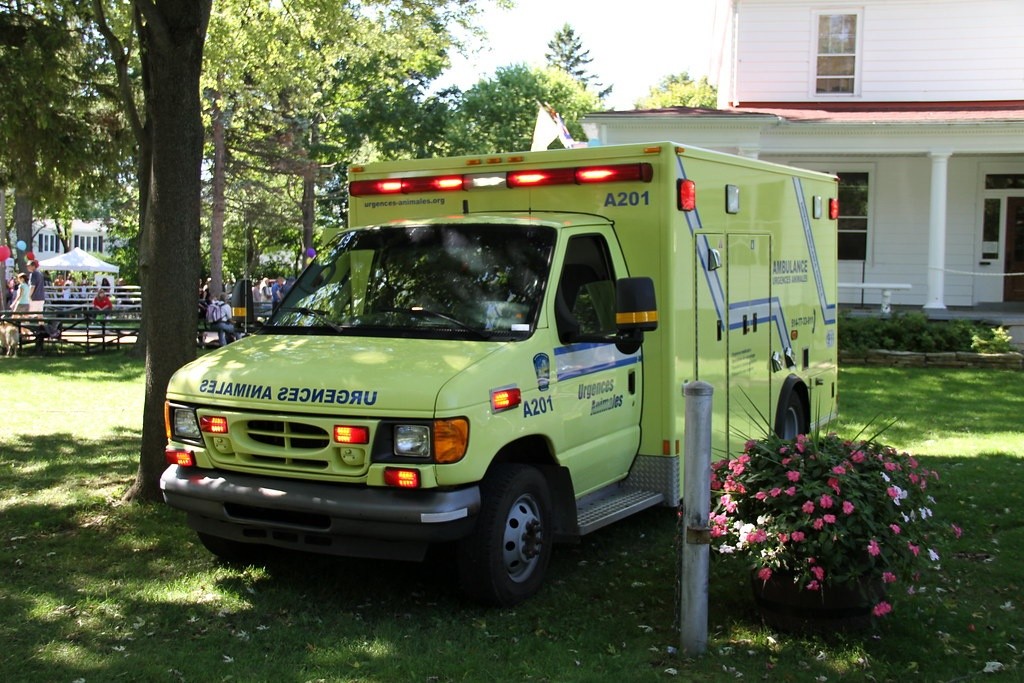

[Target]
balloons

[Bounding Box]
[17,240,27,250]
[24,255,28,261]
[0,245,11,262]
[27,251,35,259]
[27,259,40,267]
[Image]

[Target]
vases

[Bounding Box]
[753,572,869,635]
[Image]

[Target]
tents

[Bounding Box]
[36,246,120,313]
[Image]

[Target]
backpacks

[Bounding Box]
[205,302,227,324]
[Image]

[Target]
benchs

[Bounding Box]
[42,285,142,311]
[837,280,915,318]
[12,313,246,355]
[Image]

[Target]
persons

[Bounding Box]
[99,278,111,297]
[90,289,112,325]
[197,273,298,347]
[27,259,46,337]
[9,273,30,341]
[114,277,132,309]
[4,268,88,307]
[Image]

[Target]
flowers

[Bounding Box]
[707,436,942,617]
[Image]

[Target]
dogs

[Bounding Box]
[0,325,19,358]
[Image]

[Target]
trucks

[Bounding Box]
[159,140,841,601]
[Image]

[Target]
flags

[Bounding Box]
[551,109,574,148]
[530,106,558,151]
